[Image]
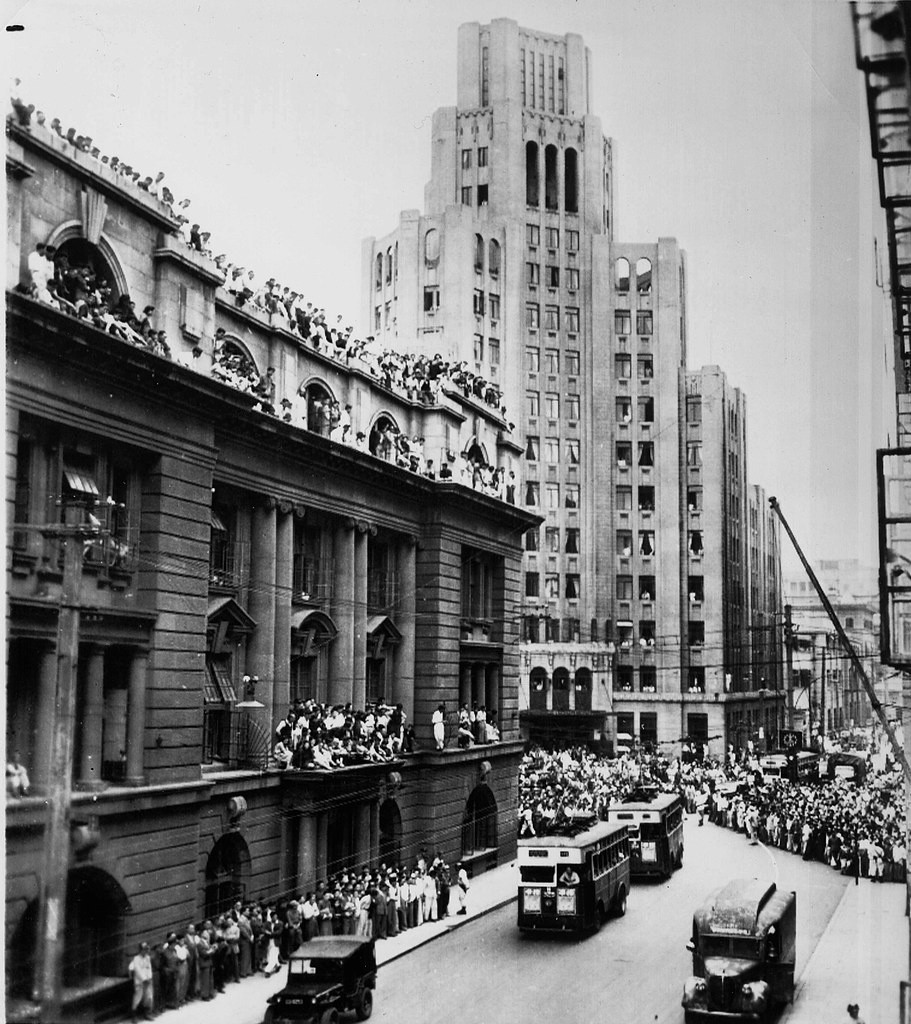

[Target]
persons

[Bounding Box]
[9,748,34,799]
[518,722,910,883]
[431,703,447,748]
[129,849,471,1021]
[456,705,507,745]
[275,694,408,768]
[4,86,514,502]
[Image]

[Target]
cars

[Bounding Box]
[263,932,379,1024]
[682,878,798,1024]
[706,960,711,963]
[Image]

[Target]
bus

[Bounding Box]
[605,763,684,883]
[516,789,631,939]
[758,750,819,786]
